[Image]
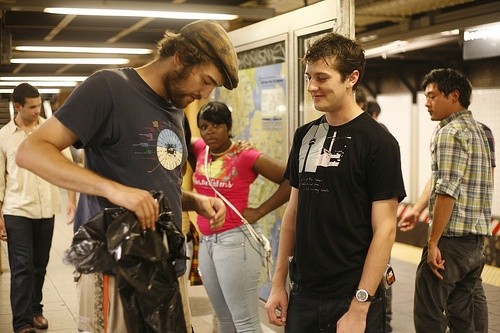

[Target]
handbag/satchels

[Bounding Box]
[259,234,271,280]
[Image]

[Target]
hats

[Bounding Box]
[179,19,239,90]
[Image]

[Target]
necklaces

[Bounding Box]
[29,127,36,134]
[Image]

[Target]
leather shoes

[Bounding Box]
[32,315,48,328]
[16,324,35,333]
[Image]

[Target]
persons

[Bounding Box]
[414,68,494,333]
[178,115,194,333]
[189,102,291,333]
[355,90,380,118]
[15,20,239,333]
[398,123,496,333]
[0,82,77,333]
[265,34,406,333]
[74,192,128,333]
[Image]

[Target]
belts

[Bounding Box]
[186,233,193,242]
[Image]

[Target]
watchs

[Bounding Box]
[354,289,374,302]
